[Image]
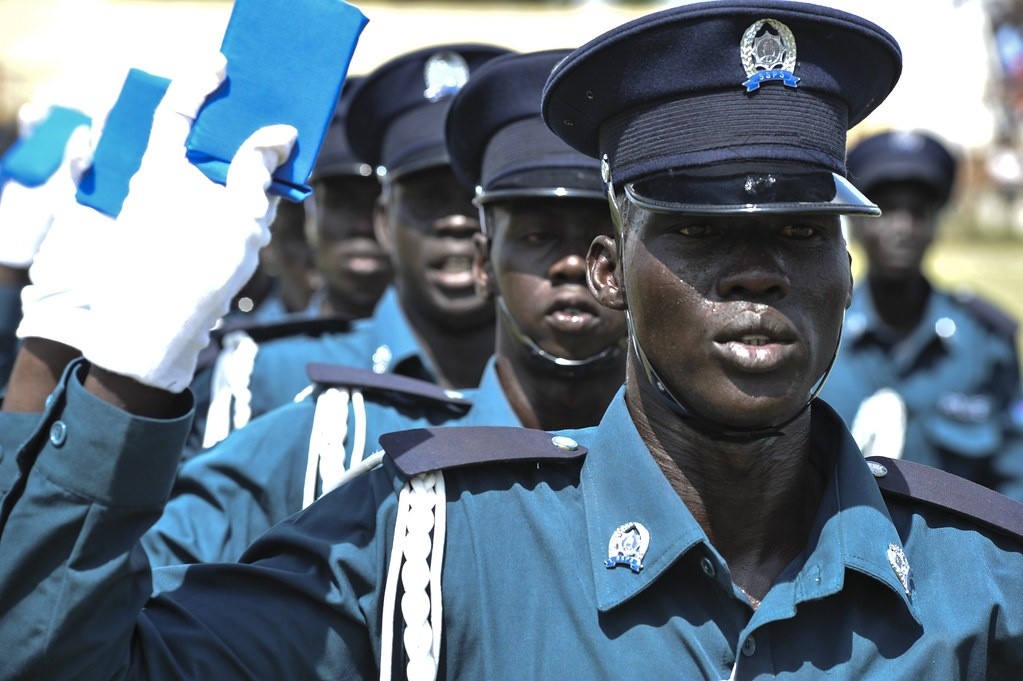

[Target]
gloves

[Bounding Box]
[0,92,94,267]
[81,50,298,396]
[17,64,174,362]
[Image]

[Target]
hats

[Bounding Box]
[444,48,608,202]
[344,42,521,182]
[846,130,956,202]
[307,78,378,180]
[540,0,901,212]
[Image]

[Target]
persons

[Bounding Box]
[0,0,1022,681]
